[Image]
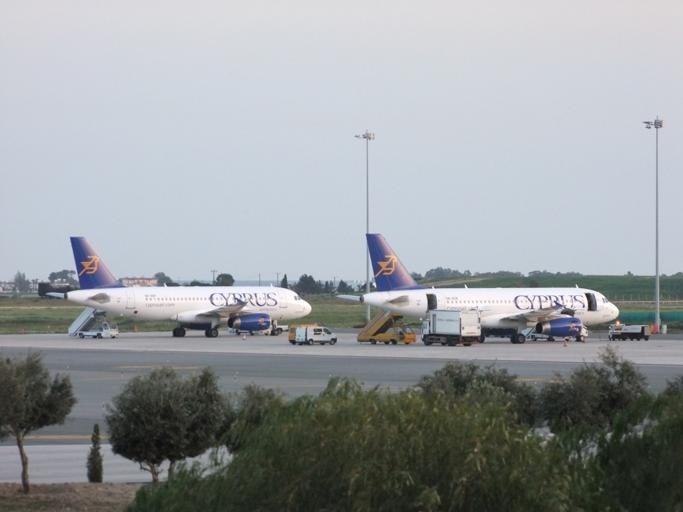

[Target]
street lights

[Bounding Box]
[353,130,375,326]
[642,113,664,334]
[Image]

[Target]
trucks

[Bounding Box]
[420,308,482,347]
[608,323,651,341]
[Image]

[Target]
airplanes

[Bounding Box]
[334,231,620,344]
[43,234,313,338]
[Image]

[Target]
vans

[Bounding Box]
[289,322,337,346]
